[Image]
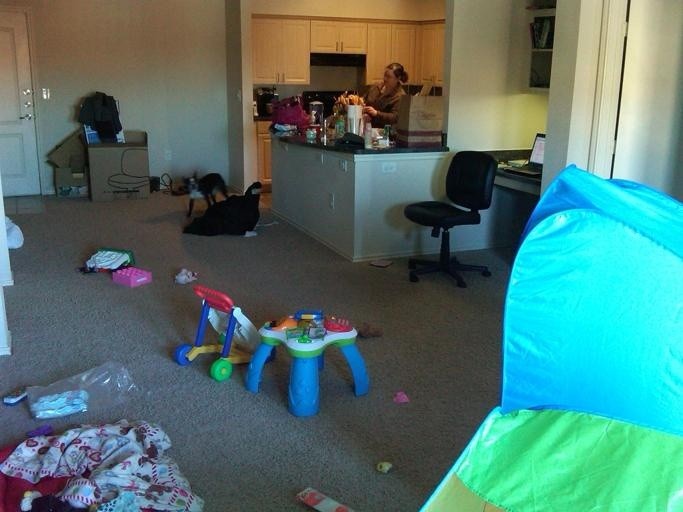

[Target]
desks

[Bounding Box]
[494,161,542,196]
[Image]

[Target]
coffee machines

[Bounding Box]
[255,86,279,117]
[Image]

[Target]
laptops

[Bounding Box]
[504,133,546,177]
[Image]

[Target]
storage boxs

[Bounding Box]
[397,95,442,148]
[47,127,150,201]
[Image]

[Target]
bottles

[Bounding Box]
[308,101,324,125]
[320,103,392,150]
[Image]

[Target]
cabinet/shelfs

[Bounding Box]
[368,20,416,86]
[310,18,368,54]
[419,24,445,87]
[522,8,556,91]
[250,19,310,84]
[257,121,272,193]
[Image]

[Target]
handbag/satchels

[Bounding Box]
[274,96,310,129]
[395,93,447,148]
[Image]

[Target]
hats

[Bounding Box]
[334,132,364,150]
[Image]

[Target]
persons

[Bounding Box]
[358,62,409,128]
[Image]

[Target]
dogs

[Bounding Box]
[182,171,229,218]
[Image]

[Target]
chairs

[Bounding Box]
[405,151,497,288]
